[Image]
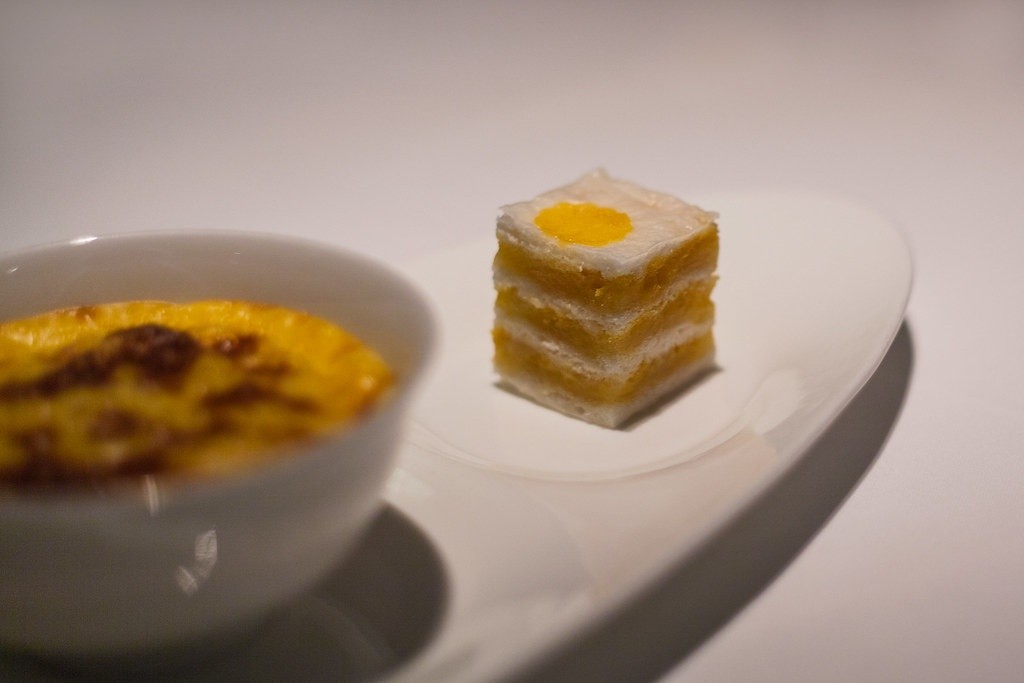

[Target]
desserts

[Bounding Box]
[492,166,719,422]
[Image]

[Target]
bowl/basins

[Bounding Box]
[2,230,438,662]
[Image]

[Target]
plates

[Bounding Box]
[0,194,914,683]
